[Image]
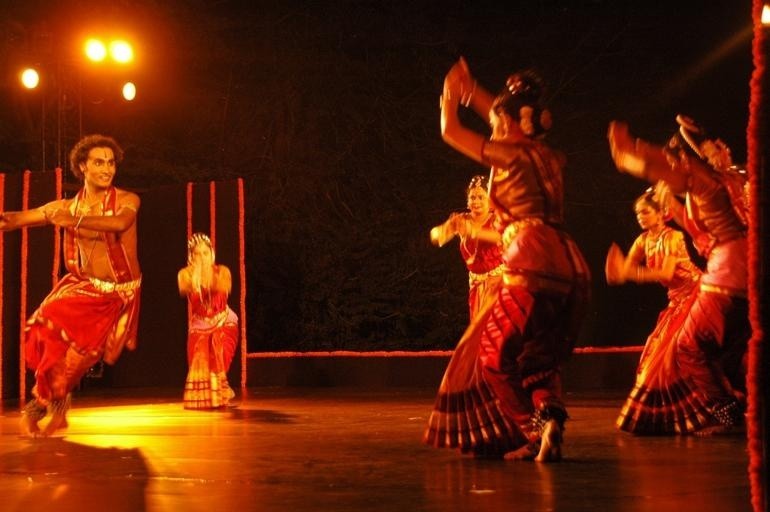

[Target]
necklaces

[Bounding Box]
[648,230,664,251]
[78,188,102,274]
[463,234,479,267]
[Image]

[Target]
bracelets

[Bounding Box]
[629,137,640,157]
[615,146,627,174]
[459,78,479,108]
[634,265,645,285]
[438,85,460,108]
[74,212,84,230]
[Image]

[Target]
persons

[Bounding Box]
[605,181,718,437]
[429,175,506,325]
[424,56,592,463]
[1,135,145,440]
[605,114,746,437]
[177,232,240,409]
